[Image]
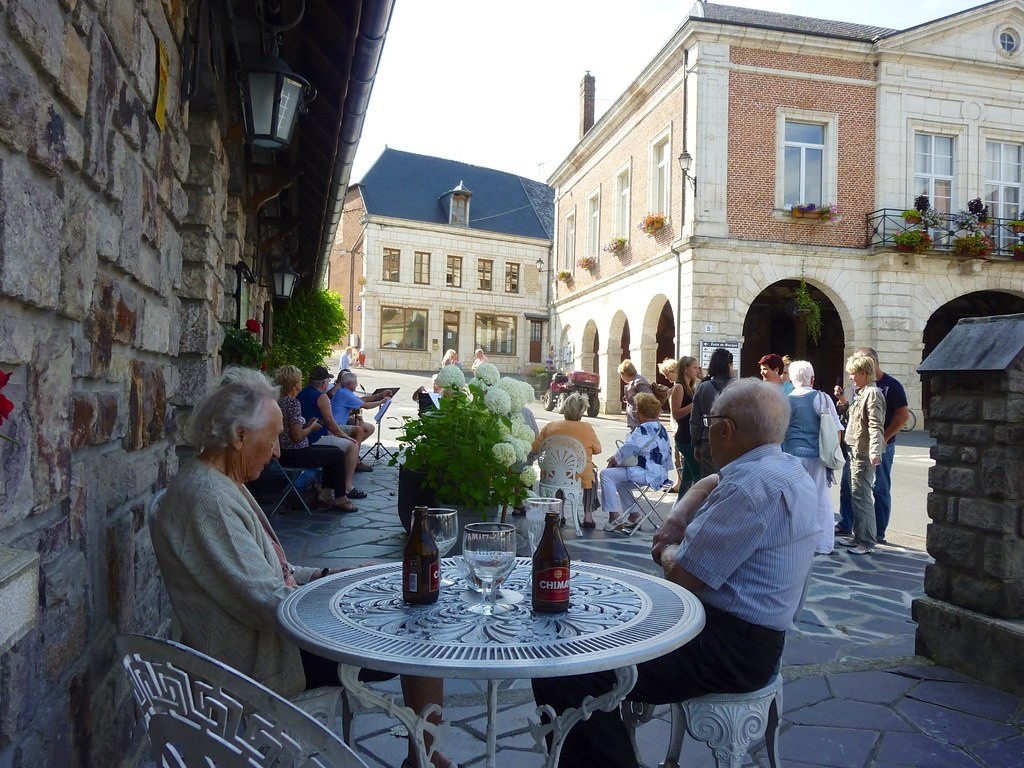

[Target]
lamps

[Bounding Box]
[678,150,697,197]
[535,257,554,273]
[221,0,321,151]
[268,254,304,303]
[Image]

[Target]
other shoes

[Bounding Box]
[815,550,840,557]
[877,535,887,543]
[356,462,372,472]
[839,538,858,546]
[833,526,853,536]
[847,548,875,554]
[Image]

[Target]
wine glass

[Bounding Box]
[410,508,458,586]
[462,521,517,616]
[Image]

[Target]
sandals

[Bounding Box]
[317,494,334,507]
[333,501,358,512]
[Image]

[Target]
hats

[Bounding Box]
[309,366,334,378]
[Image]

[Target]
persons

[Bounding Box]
[473,350,488,377]
[835,347,909,543]
[835,355,886,551]
[153,370,462,768]
[276,364,359,512]
[599,394,673,532]
[412,374,441,408]
[532,376,823,768]
[297,366,392,498]
[658,357,709,500]
[759,355,794,394]
[442,350,462,369]
[339,348,352,369]
[546,352,554,388]
[619,359,653,432]
[782,362,845,553]
[512,407,539,515]
[532,393,602,526]
[690,349,732,478]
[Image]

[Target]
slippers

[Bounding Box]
[663,489,675,493]
[582,519,596,527]
[346,487,367,498]
[561,517,566,525]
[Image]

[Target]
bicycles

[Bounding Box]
[898,409,917,432]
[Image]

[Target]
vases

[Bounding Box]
[1014,250,1024,261]
[395,459,499,558]
[560,273,571,281]
[607,242,625,254]
[953,238,995,257]
[792,208,832,219]
[650,220,664,231]
[218,333,258,370]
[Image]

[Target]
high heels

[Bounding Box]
[605,521,624,533]
[624,515,642,530]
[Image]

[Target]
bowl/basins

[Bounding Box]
[453,554,518,593]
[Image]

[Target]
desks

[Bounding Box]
[279,555,708,768]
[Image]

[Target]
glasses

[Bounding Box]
[701,414,737,429]
[323,380,331,382]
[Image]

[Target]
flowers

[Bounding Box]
[790,201,843,224]
[226,314,272,372]
[892,230,934,253]
[575,256,597,270]
[1007,241,1024,254]
[636,210,667,239]
[897,242,918,254]
[386,359,552,523]
[554,271,571,281]
[951,228,995,263]
[601,235,628,252]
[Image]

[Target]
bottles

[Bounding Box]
[531,510,571,613]
[402,507,441,605]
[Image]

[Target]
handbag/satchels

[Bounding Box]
[817,390,846,469]
[636,382,672,407]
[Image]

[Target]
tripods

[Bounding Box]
[360,387,399,460]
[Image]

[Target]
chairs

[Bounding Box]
[623,559,815,768]
[538,434,588,538]
[609,477,673,537]
[112,632,371,768]
[268,455,320,518]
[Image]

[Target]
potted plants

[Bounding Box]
[532,368,552,392]
[1006,220,1024,233]
[902,208,922,224]
[978,217,993,226]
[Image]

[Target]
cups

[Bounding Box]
[525,498,563,591]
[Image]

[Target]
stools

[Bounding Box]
[239,682,362,768]
